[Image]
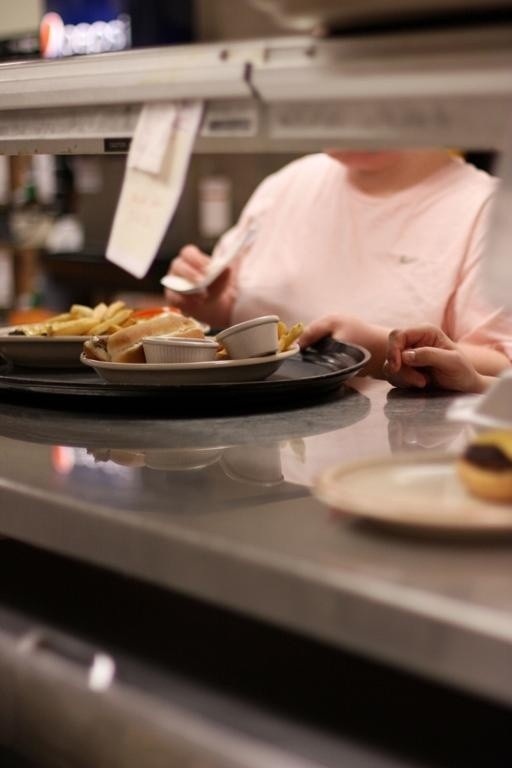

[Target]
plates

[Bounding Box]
[0,321,211,364]
[79,340,301,388]
[315,446,512,535]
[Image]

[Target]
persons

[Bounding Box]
[160,135,512,380]
[382,384,471,458]
[379,322,502,394]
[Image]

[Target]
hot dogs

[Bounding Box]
[457,428,512,502]
[84,312,203,363]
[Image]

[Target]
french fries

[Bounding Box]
[277,321,303,352]
[10,301,136,335]
